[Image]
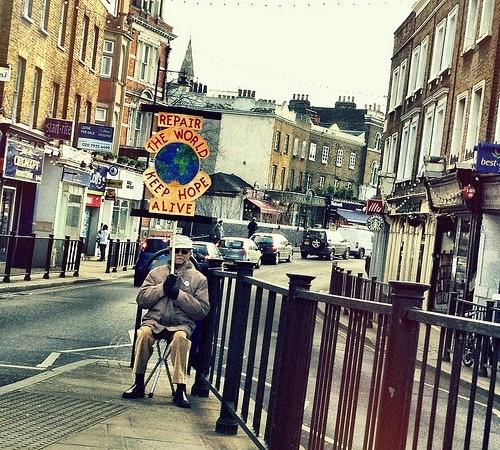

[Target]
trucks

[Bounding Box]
[337,227,375,259]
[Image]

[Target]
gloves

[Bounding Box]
[163,274,179,300]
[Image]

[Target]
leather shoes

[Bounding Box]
[122,383,145,399]
[173,390,190,408]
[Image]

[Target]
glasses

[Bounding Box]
[175,248,190,255]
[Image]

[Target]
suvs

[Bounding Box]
[249,232,295,265]
[299,228,352,261]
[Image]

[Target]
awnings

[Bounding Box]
[336,208,369,224]
[247,198,283,215]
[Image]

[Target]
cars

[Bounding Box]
[190,240,225,271]
[131,233,192,288]
[215,236,264,269]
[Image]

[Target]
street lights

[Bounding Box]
[132,57,192,264]
[305,188,316,228]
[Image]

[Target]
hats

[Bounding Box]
[167,235,194,249]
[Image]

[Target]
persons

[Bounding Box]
[248,217,258,240]
[212,220,224,244]
[123,235,211,408]
[98,225,110,261]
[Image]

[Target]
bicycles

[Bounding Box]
[458,311,498,368]
